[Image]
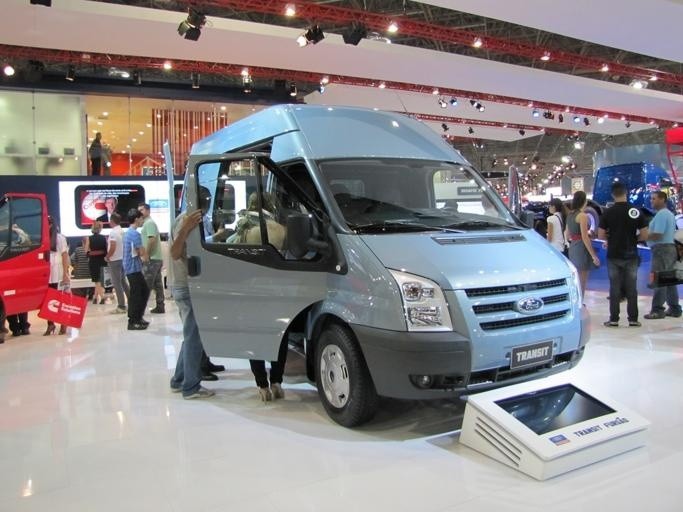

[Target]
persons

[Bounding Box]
[97,197,126,228]
[481,191,499,218]
[598,182,649,327]
[89,132,102,175]
[200,214,234,381]
[566,191,600,298]
[644,191,682,319]
[0,202,165,343]
[547,198,570,256]
[233,191,289,404]
[166,186,214,400]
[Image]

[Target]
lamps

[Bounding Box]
[59,59,302,101]
[177,2,209,42]
[296,22,324,48]
[342,21,369,46]
[430,94,591,142]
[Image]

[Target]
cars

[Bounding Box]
[518,195,603,239]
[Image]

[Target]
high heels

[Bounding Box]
[259,382,284,407]
[42,324,66,336]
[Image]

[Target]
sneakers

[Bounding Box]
[643,310,665,320]
[12,328,30,336]
[127,317,150,330]
[111,307,126,315]
[664,307,681,318]
[150,303,165,313]
[171,379,215,400]
[628,320,642,328]
[604,319,619,327]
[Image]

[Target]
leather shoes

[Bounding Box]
[200,361,225,380]
[92,298,105,304]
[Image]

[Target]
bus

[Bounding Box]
[471,166,519,216]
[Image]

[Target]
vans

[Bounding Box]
[594,162,679,220]
[1,191,52,336]
[163,102,592,429]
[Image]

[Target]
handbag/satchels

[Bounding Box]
[37,288,89,329]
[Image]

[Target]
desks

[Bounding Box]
[0,154,81,176]
